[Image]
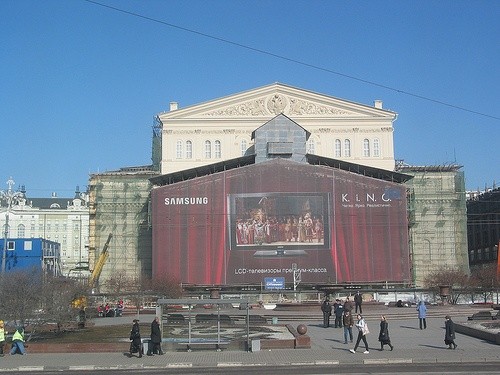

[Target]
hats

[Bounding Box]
[133,318,139,322]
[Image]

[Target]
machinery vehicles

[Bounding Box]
[70,232,114,311]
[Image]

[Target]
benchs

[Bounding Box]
[178,341,231,351]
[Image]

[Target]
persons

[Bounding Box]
[105,303,110,317]
[354,291,363,314]
[349,314,370,353]
[147,317,167,356]
[127,318,142,358]
[97,306,104,313]
[444,316,458,350]
[0,320,8,357]
[343,310,354,344]
[417,301,426,329]
[9,322,27,355]
[334,300,343,328]
[118,298,123,308]
[378,315,394,351]
[321,300,331,328]
[344,297,354,312]
[333,299,339,305]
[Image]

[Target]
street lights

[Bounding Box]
[0,175,34,273]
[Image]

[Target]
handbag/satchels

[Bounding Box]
[361,319,370,335]
[18,327,23,333]
[445,335,449,345]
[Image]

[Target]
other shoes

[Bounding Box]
[454,344,457,348]
[391,346,393,351]
[448,347,452,349]
[364,350,369,353]
[350,348,355,353]
[379,348,384,351]
